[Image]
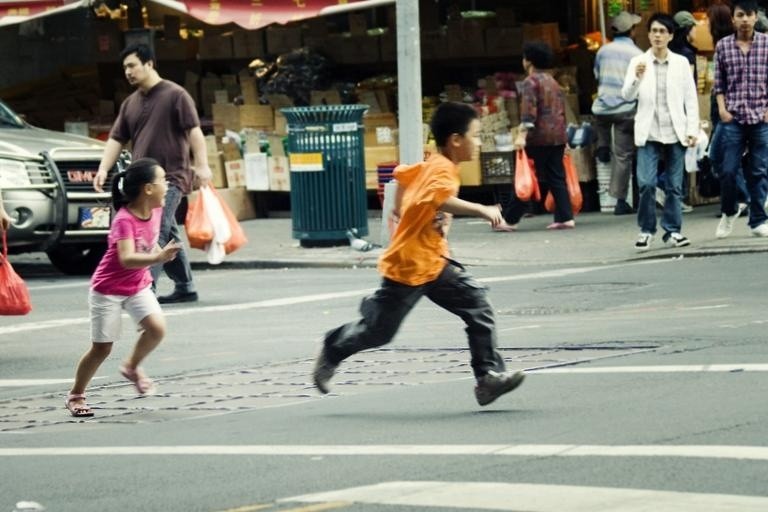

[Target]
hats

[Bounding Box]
[671,10,698,29]
[607,11,642,35]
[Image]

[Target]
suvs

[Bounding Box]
[1,99,134,278]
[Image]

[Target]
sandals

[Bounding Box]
[119,364,154,394]
[64,391,96,417]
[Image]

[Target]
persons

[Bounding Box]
[588,11,645,216]
[621,12,701,253]
[0,201,10,232]
[313,101,527,407]
[92,41,214,305]
[492,41,577,232]
[654,10,701,215]
[705,2,751,217]
[64,158,185,417]
[712,1,768,240]
[753,7,768,209]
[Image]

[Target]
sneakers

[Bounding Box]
[473,367,527,407]
[633,232,654,252]
[310,345,340,395]
[748,220,767,239]
[681,202,695,214]
[157,288,202,304]
[714,205,743,239]
[662,231,693,250]
[598,148,612,164]
[613,203,636,216]
[655,187,666,208]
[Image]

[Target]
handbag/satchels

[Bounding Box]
[683,128,716,175]
[697,155,722,198]
[590,93,639,123]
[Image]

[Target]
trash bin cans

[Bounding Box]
[281,103,373,248]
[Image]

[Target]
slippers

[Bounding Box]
[544,219,578,232]
[491,222,519,233]
